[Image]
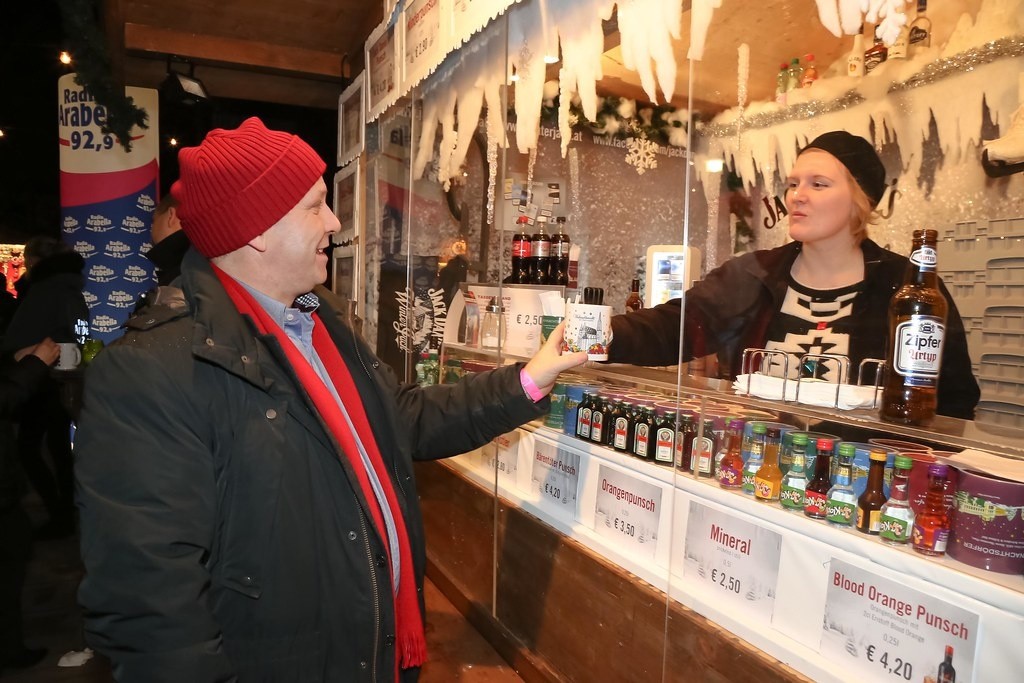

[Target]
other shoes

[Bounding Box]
[1,647,48,668]
[33,518,75,541]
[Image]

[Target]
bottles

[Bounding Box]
[878,227,948,427]
[423,348,440,386]
[575,390,784,504]
[856,448,888,533]
[415,352,428,385]
[625,278,643,313]
[774,53,818,99]
[480,295,507,351]
[444,359,461,385]
[878,455,915,543]
[780,433,810,511]
[848,0,932,79]
[826,444,857,523]
[911,461,950,556]
[512,216,530,284]
[549,216,570,285]
[803,438,834,519]
[530,216,550,284]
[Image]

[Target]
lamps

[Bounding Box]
[157,59,211,117]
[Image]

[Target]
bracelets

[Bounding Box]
[519,368,542,403]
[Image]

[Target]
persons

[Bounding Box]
[75,117,589,683]
[605,131,981,419]
[0,195,193,668]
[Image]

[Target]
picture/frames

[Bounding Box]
[403,0,523,97]
[365,4,404,123]
[337,70,366,168]
[332,157,361,244]
[333,243,358,317]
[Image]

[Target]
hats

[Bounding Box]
[171,116,327,258]
[797,131,887,210]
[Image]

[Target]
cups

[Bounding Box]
[82,339,103,363]
[561,303,612,362]
[54,342,82,370]
